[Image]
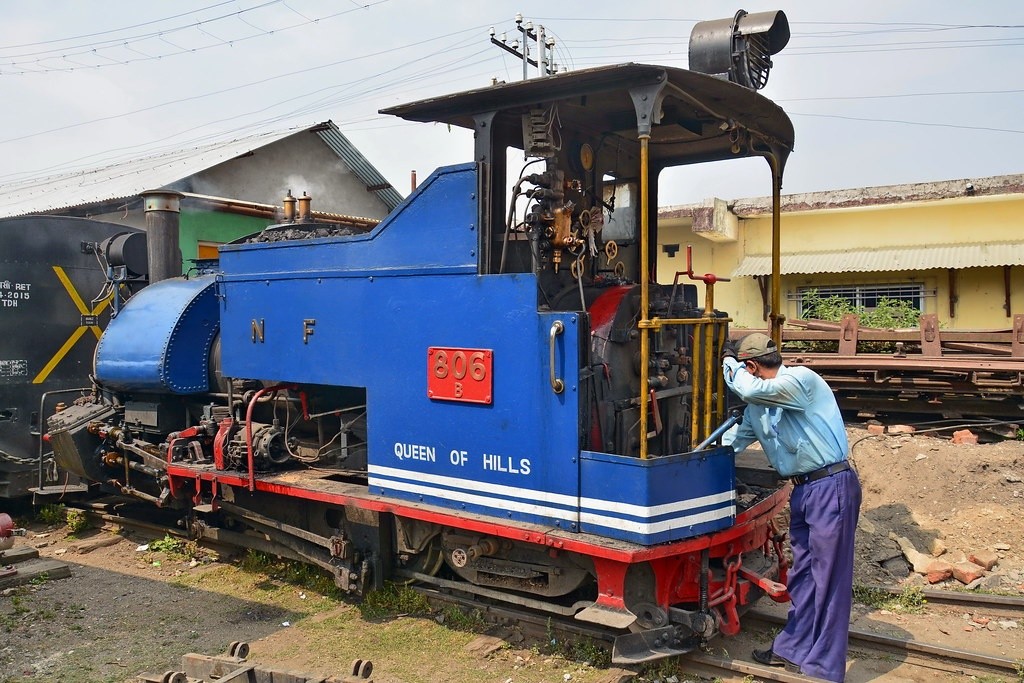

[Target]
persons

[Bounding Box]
[721,334,863,683]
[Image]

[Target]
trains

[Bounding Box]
[30,4,795,665]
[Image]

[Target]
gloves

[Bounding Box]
[722,340,737,360]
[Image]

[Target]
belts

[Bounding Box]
[791,461,850,486]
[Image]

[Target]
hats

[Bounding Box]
[734,333,778,360]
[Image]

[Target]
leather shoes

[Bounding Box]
[752,649,801,673]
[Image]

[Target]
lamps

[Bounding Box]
[965,183,974,197]
